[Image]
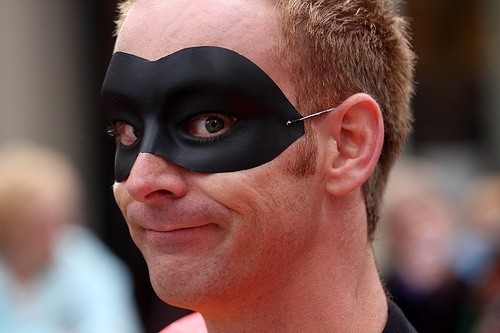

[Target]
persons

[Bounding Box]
[0,145,500,333]
[99,0,417,333]
